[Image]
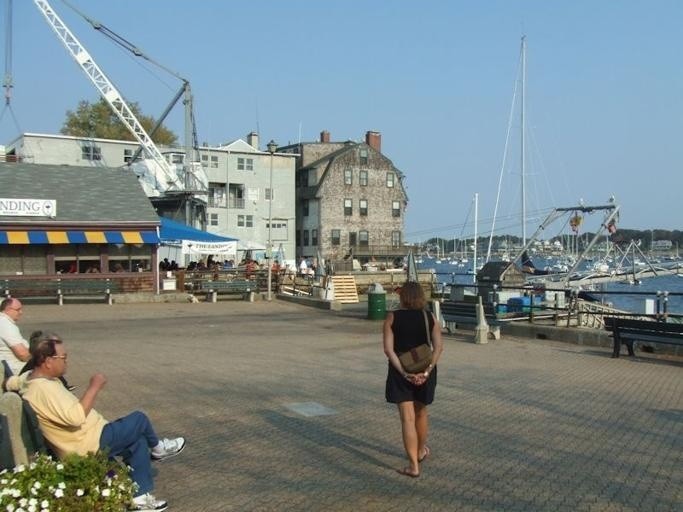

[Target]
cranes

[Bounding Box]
[0,0,210,202]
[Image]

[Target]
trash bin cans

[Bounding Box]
[367,282,387,321]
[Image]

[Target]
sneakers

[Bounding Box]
[66,384,77,390]
[150,437,186,461]
[128,492,169,512]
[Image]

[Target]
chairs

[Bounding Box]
[0,357,126,480]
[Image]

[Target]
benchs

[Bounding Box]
[197,281,262,301]
[0,279,121,304]
[427,298,507,346]
[603,315,683,358]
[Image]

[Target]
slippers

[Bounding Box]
[395,467,419,477]
[418,445,430,463]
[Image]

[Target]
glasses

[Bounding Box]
[52,354,67,359]
[8,307,21,311]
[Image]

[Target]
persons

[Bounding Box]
[383,282,443,477]
[56,256,332,289]
[5,333,186,512]
[0,297,77,391]
[17,329,81,391]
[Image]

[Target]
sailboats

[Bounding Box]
[634,240,681,266]
[476,35,613,272]
[416,236,468,267]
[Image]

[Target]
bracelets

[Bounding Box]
[428,364,434,369]
[423,371,429,377]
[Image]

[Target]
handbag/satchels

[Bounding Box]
[399,343,433,373]
